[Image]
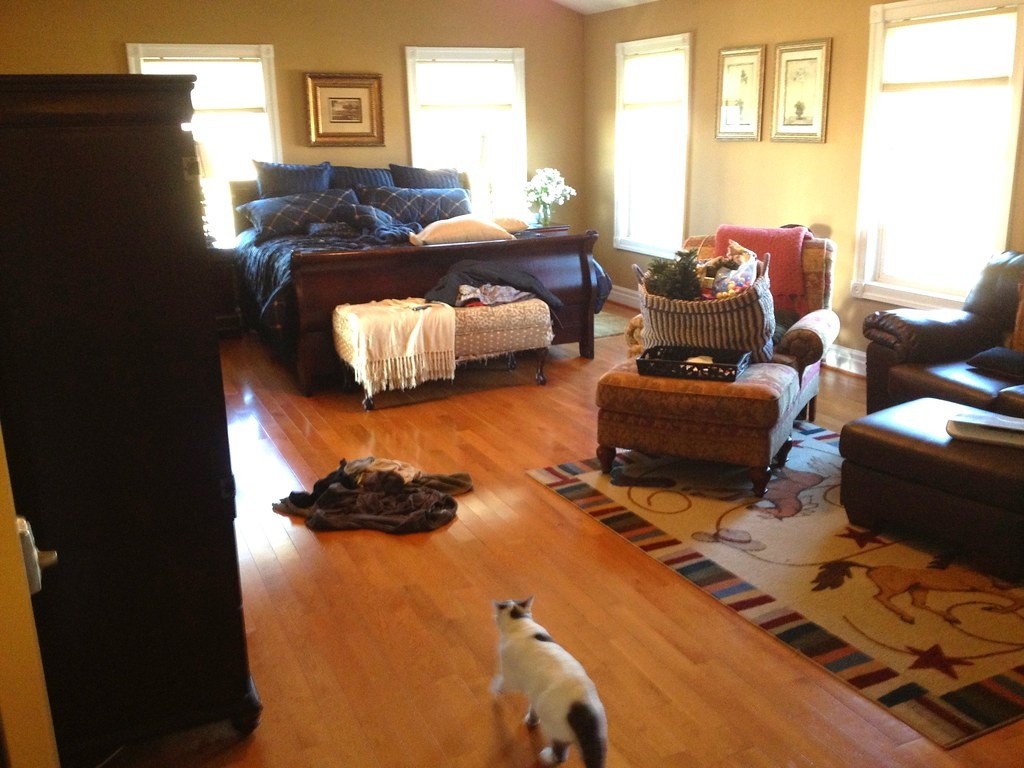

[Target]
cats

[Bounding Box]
[488,591,608,768]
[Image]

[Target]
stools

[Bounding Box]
[839,397,1024,582]
[596,359,799,498]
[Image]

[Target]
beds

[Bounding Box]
[229,169,599,397]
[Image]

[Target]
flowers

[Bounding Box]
[528,168,577,205]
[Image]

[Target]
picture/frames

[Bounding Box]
[303,72,385,148]
[715,44,766,142]
[770,37,832,143]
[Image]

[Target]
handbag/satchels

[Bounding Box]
[633,253,774,362]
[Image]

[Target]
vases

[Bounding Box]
[538,202,550,224]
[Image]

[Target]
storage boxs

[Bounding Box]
[637,344,751,381]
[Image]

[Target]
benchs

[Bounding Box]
[331,293,553,409]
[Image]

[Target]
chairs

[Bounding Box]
[623,225,840,421]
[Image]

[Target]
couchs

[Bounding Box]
[863,251,1024,418]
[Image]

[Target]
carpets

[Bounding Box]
[593,311,629,337]
[524,416,1024,747]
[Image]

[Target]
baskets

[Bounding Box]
[637,346,752,382]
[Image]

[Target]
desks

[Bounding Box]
[526,225,570,236]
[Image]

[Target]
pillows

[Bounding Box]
[410,213,517,245]
[355,184,473,225]
[389,164,462,189]
[330,166,395,191]
[966,346,1024,383]
[236,187,360,246]
[493,214,529,233]
[252,160,331,198]
[1012,283,1024,352]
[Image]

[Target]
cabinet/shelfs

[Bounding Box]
[0,73,264,749]
